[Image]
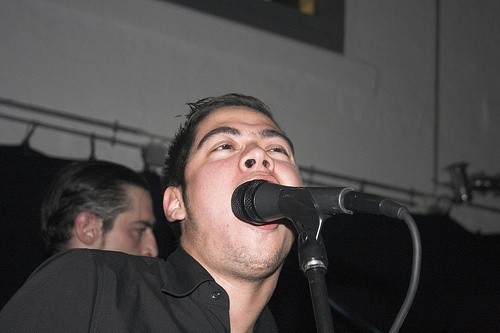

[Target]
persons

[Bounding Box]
[41,160,159,258]
[0,92,304,333]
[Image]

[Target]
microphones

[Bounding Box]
[231,179,410,227]
